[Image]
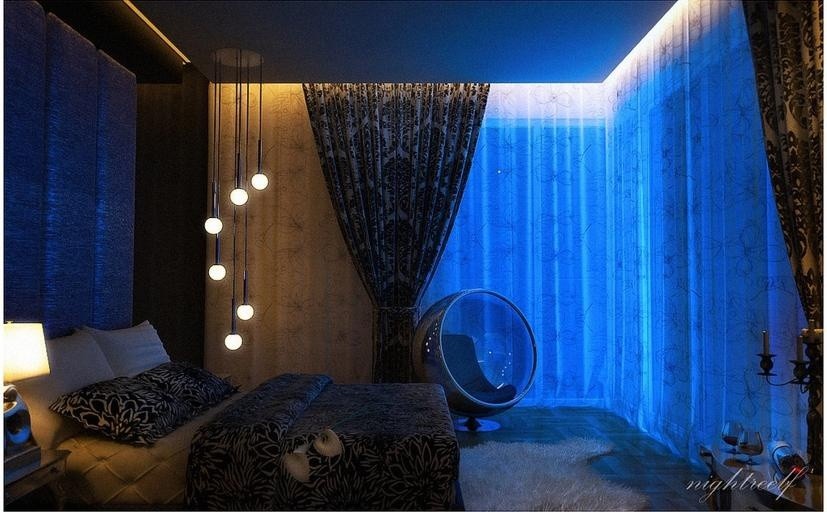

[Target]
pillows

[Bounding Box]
[14,331,118,449]
[136,359,238,414]
[83,318,172,377]
[49,375,200,447]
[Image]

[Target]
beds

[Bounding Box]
[22,322,466,509]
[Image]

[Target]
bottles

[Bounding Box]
[769,439,806,477]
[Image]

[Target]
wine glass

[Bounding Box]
[720,420,742,454]
[737,429,763,465]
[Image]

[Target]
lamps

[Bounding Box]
[206,40,269,351]
[3,320,52,446]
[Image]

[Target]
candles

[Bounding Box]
[797,335,804,360]
[809,318,814,342]
[762,329,770,354]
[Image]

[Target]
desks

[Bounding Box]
[700,435,822,511]
[5,446,71,512]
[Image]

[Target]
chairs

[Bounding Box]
[435,332,516,402]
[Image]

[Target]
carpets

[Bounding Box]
[450,432,649,512]
